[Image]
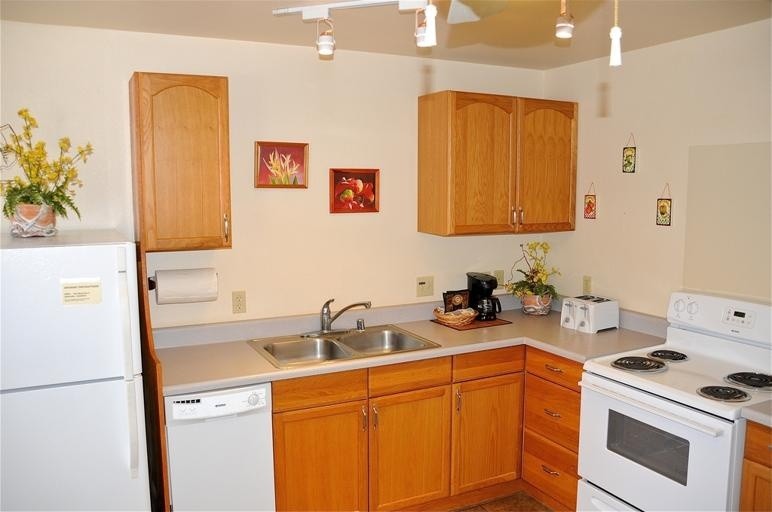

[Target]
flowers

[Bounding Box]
[0,108,94,218]
[503,240,561,302]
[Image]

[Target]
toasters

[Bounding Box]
[560,292,621,334]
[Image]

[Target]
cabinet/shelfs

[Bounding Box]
[128,71,232,252]
[583,276,591,294]
[520,344,585,512]
[740,420,772,512]
[450,344,526,512]
[417,89,578,237]
[271,355,452,512]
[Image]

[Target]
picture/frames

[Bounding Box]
[254,140,309,189]
[329,168,380,213]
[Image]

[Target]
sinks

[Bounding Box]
[263,338,349,366]
[336,329,428,355]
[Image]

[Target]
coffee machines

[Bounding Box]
[465,271,503,322]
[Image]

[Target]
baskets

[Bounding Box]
[433,307,479,327]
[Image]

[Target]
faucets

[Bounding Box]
[320,299,372,332]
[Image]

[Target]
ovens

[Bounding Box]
[577,377,747,511]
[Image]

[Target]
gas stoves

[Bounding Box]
[580,328,772,421]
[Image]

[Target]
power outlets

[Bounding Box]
[232,291,246,314]
[495,270,504,289]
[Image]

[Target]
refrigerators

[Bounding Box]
[0,228,152,512]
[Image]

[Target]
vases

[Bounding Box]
[7,204,56,238]
[521,294,553,315]
